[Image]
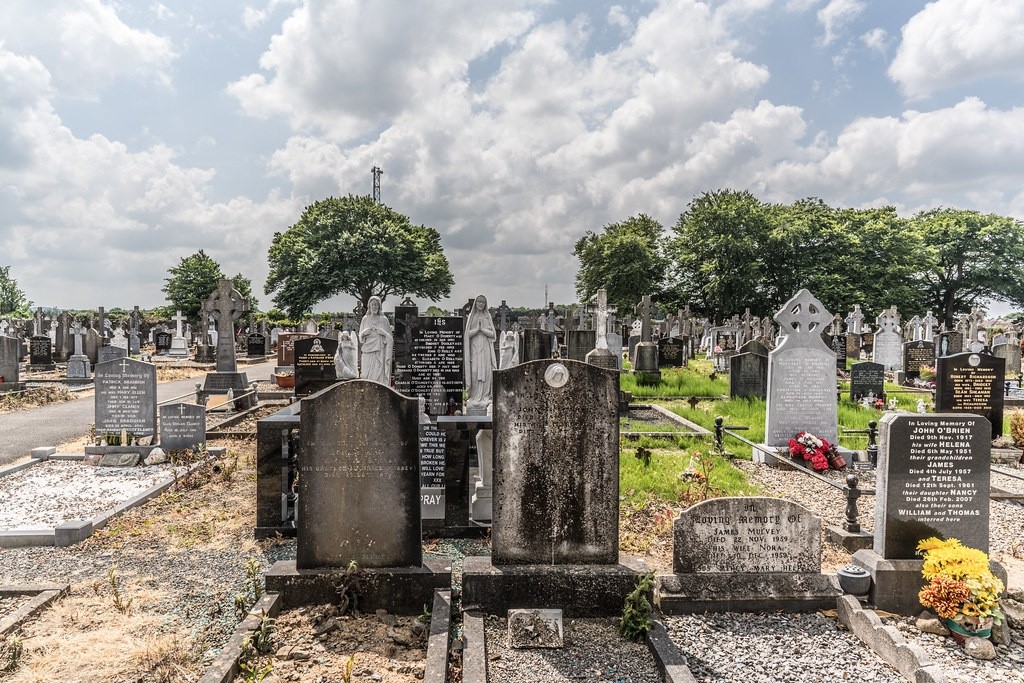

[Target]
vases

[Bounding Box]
[947,613,993,645]
[276,375,295,389]
[830,456,846,470]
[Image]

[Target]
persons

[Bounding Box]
[499,331,518,370]
[464,295,497,409]
[547,367,567,385]
[359,296,393,386]
[71,317,82,328]
[334,331,358,378]
[310,340,324,352]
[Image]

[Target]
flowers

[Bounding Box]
[914,537,1006,633]
[873,399,884,406]
[786,431,841,470]
[920,366,937,385]
[273,370,295,377]
[1009,405,1024,448]
